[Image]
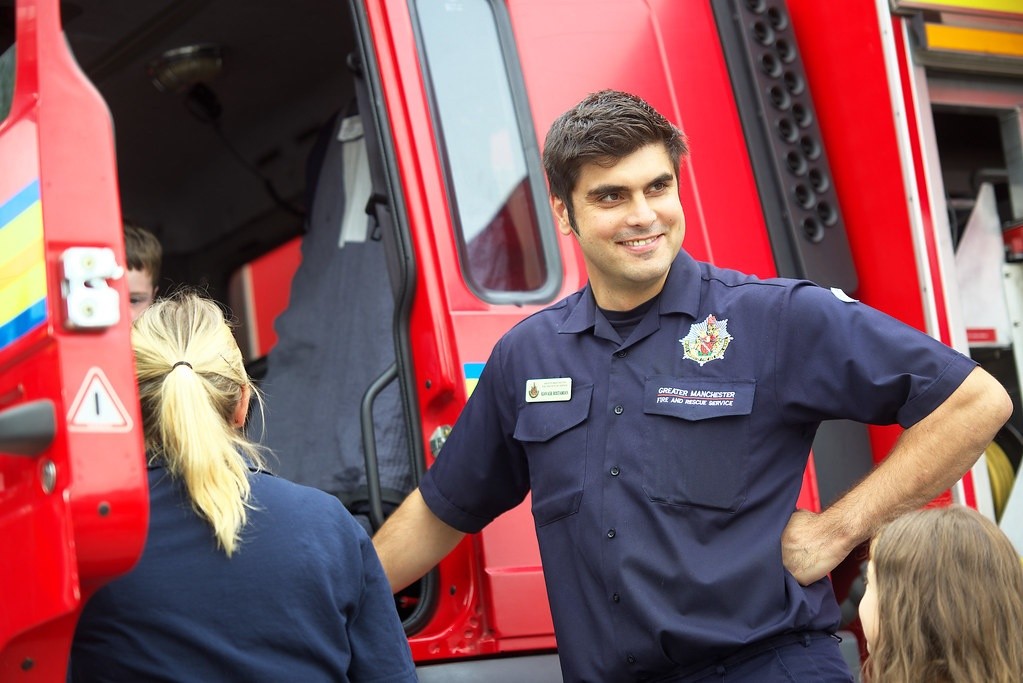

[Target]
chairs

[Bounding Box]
[227,110,413,530]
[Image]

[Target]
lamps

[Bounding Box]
[141,43,230,95]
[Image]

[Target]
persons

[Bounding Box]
[123,224,163,323]
[372,87,1014,682]
[856,505,1023,682]
[66,291,420,683]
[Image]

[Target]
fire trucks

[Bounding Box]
[0,0,1023,682]
